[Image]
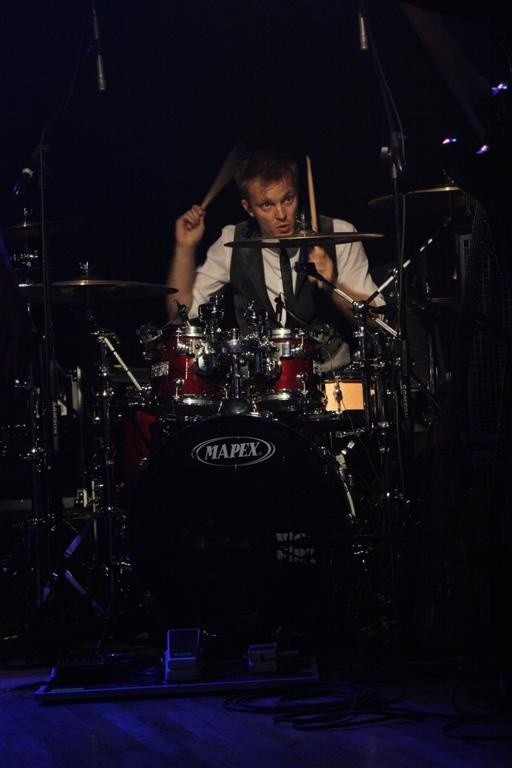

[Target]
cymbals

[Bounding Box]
[369,186,468,205]
[19,278,180,300]
[224,232,388,248]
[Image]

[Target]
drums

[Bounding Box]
[132,411,366,612]
[315,375,383,419]
[241,326,327,412]
[139,322,234,412]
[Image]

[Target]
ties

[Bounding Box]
[280,248,293,299]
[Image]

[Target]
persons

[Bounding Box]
[162,145,385,376]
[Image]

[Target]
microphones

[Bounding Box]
[88,3,107,93]
[359,1,370,52]
